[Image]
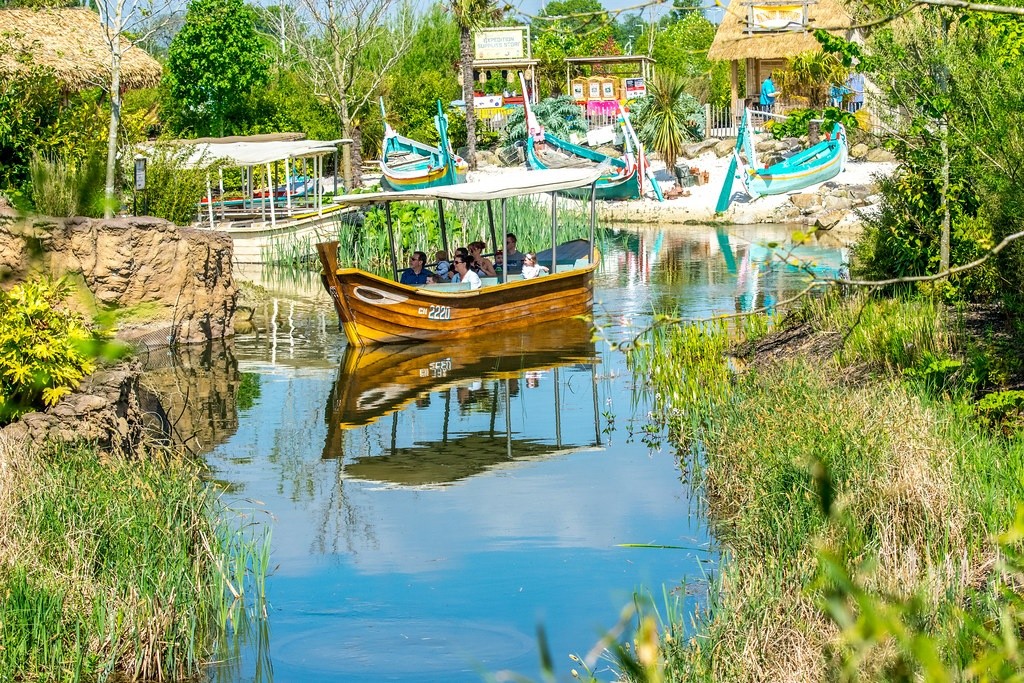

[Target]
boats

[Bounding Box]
[315,165,603,349]
[518,71,640,200]
[317,338,612,492]
[379,95,469,191]
[733,106,849,195]
[118,132,360,264]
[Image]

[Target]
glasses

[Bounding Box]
[507,241,513,245]
[410,256,417,261]
[470,249,474,254]
[455,261,464,264]
[455,254,461,257]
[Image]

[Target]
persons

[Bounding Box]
[760,73,782,121]
[830,72,864,112]
[399,251,435,284]
[426,233,549,290]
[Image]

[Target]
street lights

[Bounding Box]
[707,10,721,35]
[634,25,643,37]
